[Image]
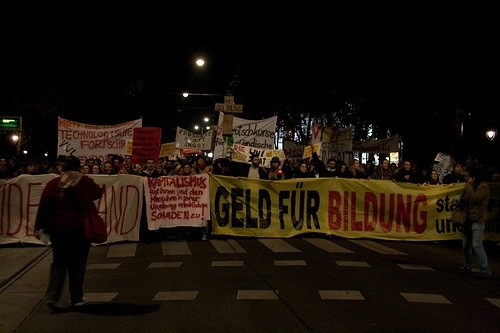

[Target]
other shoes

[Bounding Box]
[84,207,107,243]
[73,297,88,306]
[47,299,63,312]
[459,265,472,273]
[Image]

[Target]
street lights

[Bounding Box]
[194,117,211,157]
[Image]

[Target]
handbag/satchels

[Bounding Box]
[451,208,467,224]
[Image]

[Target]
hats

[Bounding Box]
[270,157,281,165]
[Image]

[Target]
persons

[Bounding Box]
[457,168,491,277]
[0,158,64,247]
[34,155,103,306]
[246,155,500,245]
[83,154,233,241]
